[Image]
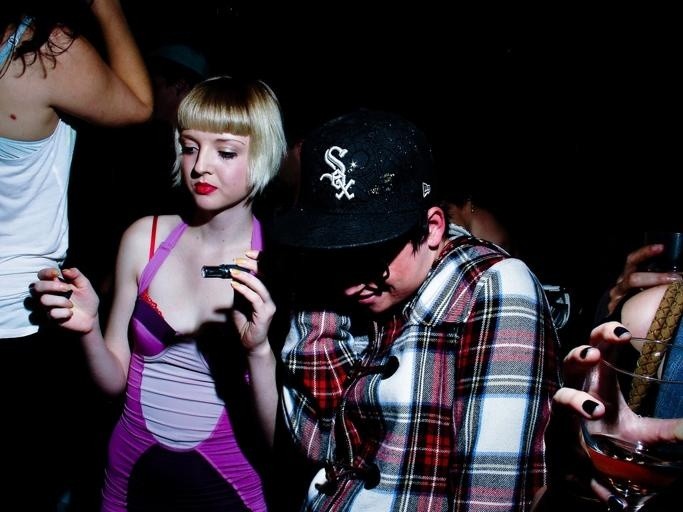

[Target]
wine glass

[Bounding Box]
[580,335,682,511]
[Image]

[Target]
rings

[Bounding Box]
[615,277,632,294]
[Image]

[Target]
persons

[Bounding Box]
[0,2,155,512]
[449,140,575,303]
[277,117,559,512]
[553,282,682,512]
[606,242,682,312]
[27,75,292,512]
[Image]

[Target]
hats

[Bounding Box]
[287,114,434,250]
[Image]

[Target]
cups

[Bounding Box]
[642,228,681,275]
